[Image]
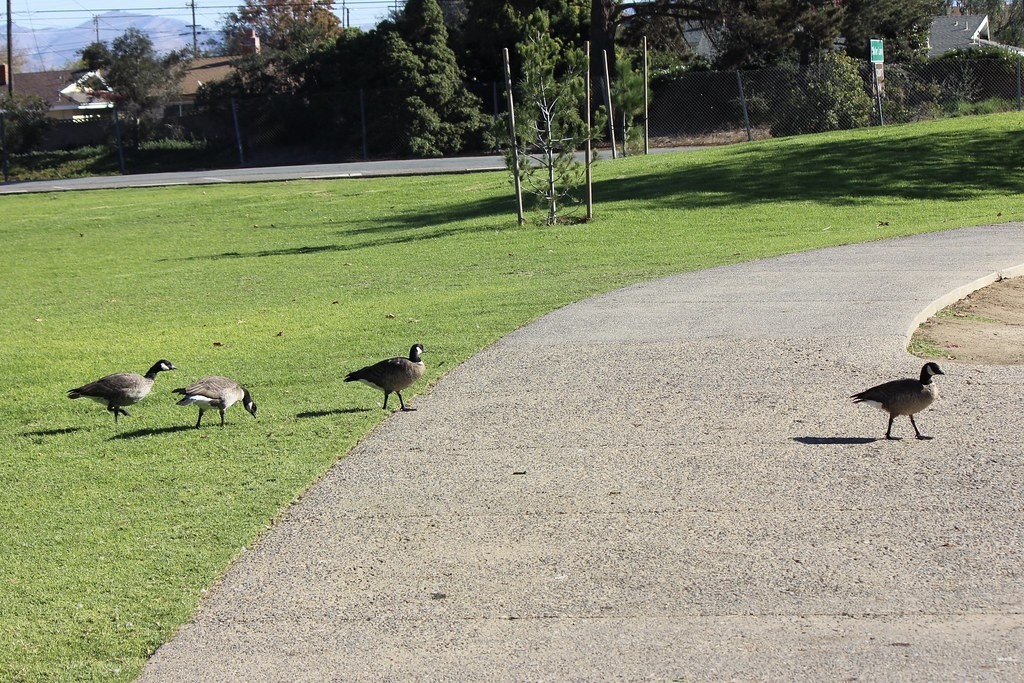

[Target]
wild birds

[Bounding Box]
[343,343,427,412]
[65,359,178,426]
[171,375,257,431]
[849,362,946,441]
